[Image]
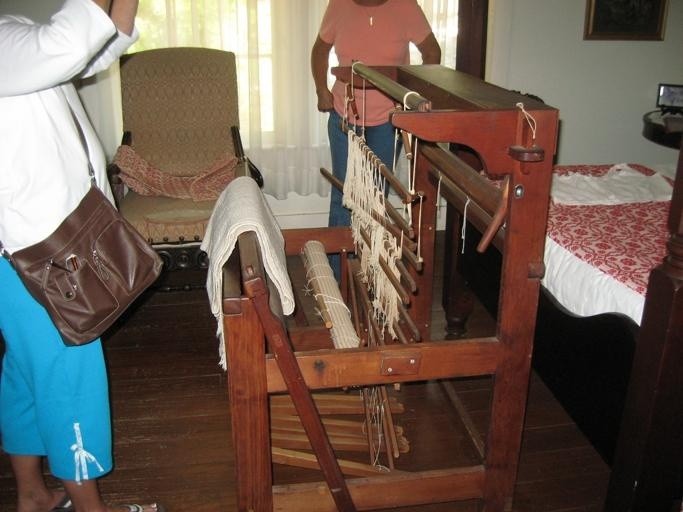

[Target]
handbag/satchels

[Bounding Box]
[8,181,166,350]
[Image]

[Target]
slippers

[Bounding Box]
[47,484,73,509]
[120,503,144,512]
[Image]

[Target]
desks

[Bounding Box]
[642,109,683,149]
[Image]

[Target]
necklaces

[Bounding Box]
[361,1,378,28]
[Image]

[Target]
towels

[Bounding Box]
[199,174,296,370]
[102,145,238,202]
[552,163,674,205]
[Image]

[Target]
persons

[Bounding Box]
[308,1,442,296]
[0,1,158,512]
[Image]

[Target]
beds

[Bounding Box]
[473,159,683,512]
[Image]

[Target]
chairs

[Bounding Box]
[108,45,263,293]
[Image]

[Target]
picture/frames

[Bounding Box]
[656,84,683,107]
[584,0,670,41]
